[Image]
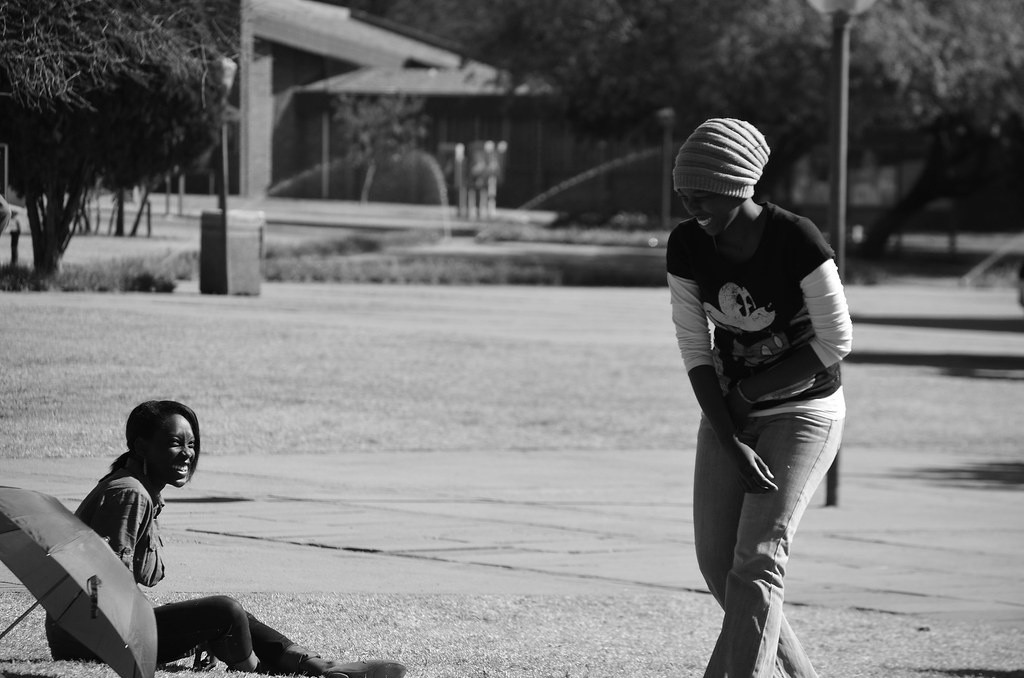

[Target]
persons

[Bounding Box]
[666,116,854,678]
[45,399,407,678]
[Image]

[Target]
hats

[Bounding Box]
[673,118,771,198]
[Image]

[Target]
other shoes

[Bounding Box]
[326,660,406,678]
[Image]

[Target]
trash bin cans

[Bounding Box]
[200,207,268,297]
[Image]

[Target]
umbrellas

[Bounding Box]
[0,483,158,678]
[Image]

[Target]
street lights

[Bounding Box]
[804,1,877,281]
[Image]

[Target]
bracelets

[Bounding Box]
[737,378,760,404]
